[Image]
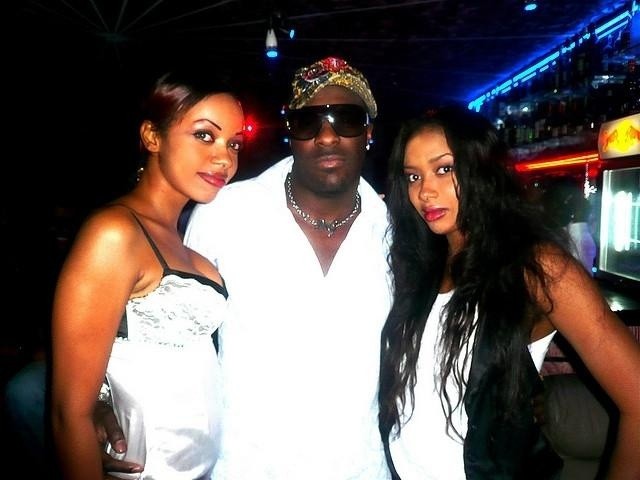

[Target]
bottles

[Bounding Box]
[473,0,639,144]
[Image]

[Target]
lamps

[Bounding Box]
[265,8,296,59]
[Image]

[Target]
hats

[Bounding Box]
[289,57,378,118]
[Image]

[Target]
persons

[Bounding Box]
[564,197,598,277]
[376,103,640,480]
[91,57,552,480]
[49,66,245,480]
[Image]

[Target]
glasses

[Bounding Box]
[284,104,370,141]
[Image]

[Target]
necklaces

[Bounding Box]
[287,173,360,237]
